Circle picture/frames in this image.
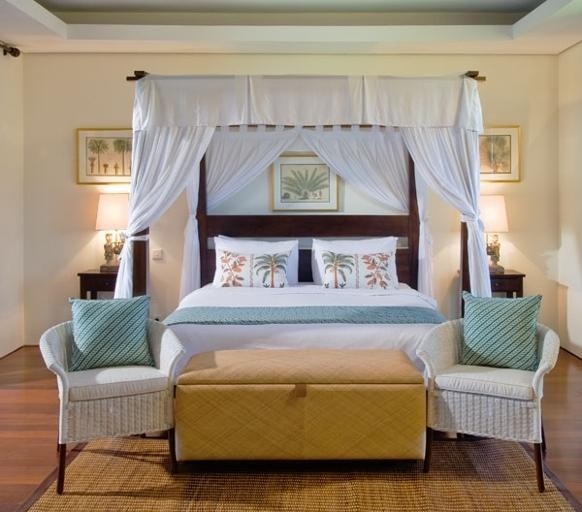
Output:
[271,155,339,212]
[479,125,521,183]
[77,128,134,185]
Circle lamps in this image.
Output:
[95,193,130,274]
[478,195,509,273]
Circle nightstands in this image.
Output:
[455,269,526,299]
[77,268,118,299]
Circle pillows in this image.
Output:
[456,290,543,371]
[212,235,296,288]
[312,235,398,292]
[68,295,156,373]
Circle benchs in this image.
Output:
[173,349,427,463]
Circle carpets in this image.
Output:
[16,436,582,512]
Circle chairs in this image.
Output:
[415,318,561,493]
[39,319,186,495]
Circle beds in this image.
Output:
[112,72,492,440]
